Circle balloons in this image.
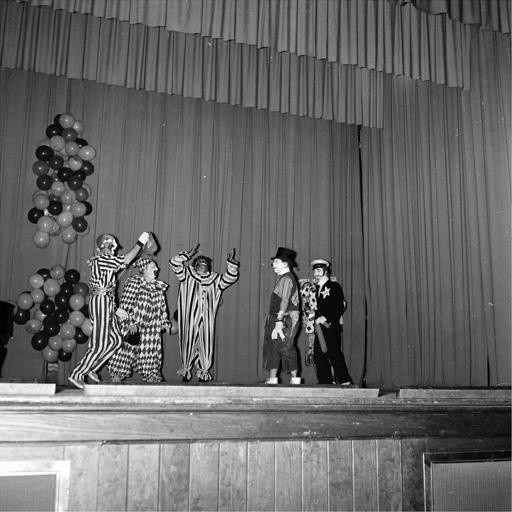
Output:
[28,112,96,249]
[9,265,92,363]
[173,308,179,320]
[165,318,178,334]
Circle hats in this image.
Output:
[310,260,331,271]
[270,246,299,268]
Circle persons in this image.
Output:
[169,243,240,383]
[68,231,150,389]
[108,258,171,383]
[310,258,352,386]
[262,246,303,385]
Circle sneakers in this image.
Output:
[257,377,280,387]
[68,377,86,389]
[198,376,208,386]
[181,372,191,385]
[88,371,100,383]
[289,377,302,385]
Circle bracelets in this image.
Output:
[277,319,283,322]
[136,241,143,247]
